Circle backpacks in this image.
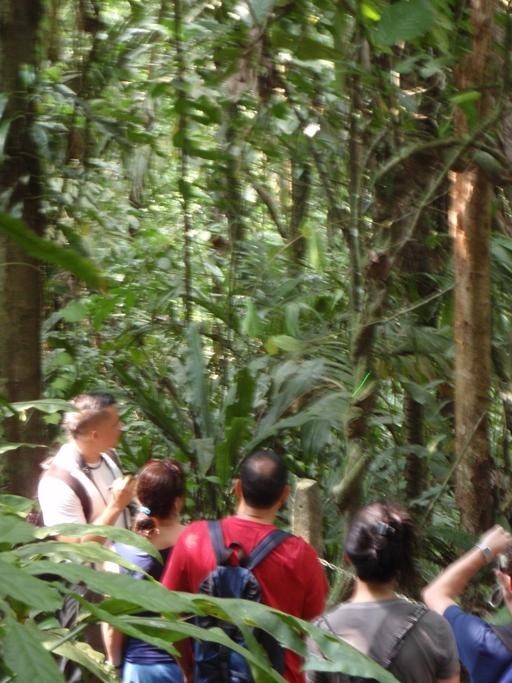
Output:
[313,604,427,683]
[21,466,90,526]
[186,520,295,683]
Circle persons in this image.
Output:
[37,392,136,683]
[159,452,331,683]
[420,524,512,683]
[304,504,459,683]
[99,459,203,683]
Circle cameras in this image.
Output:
[497,549,512,574]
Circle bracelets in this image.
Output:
[104,660,123,669]
[475,542,494,565]
